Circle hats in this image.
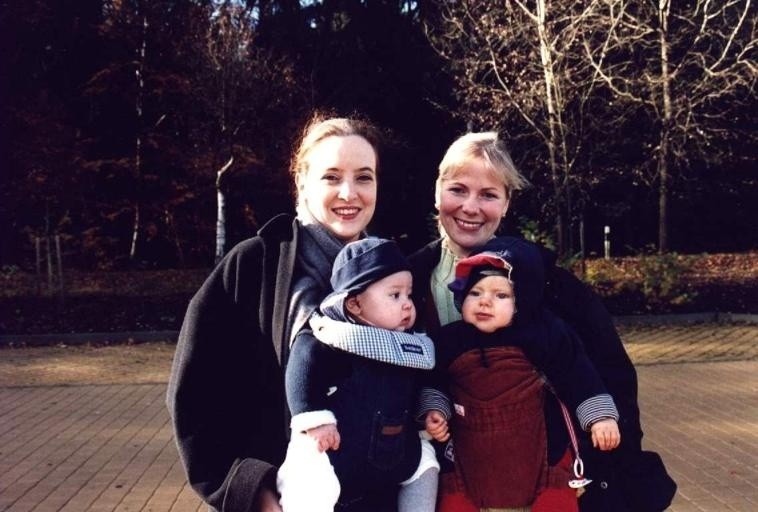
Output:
[329,237,414,294]
[447,250,525,314]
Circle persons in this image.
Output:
[275,236,444,512]
[402,131,679,512]
[166,117,394,512]
[414,234,622,512]
